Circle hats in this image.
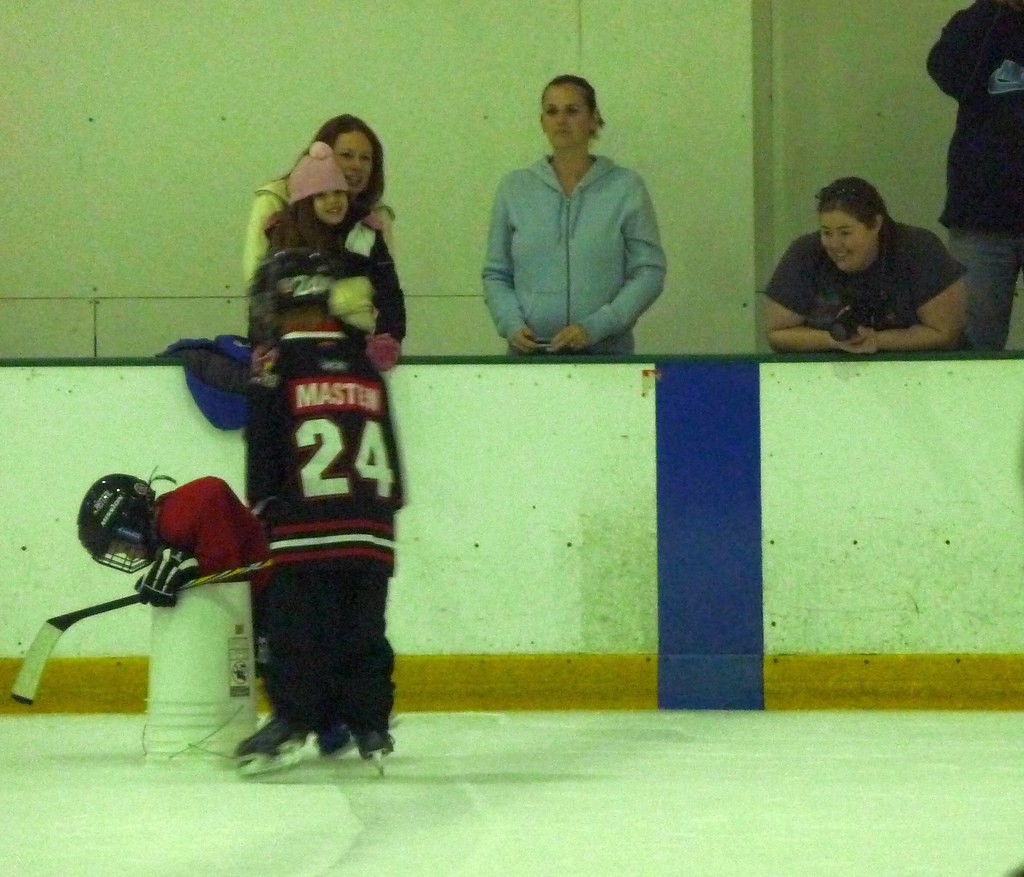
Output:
[289,140,349,205]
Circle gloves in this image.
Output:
[134,545,199,606]
[364,333,401,372]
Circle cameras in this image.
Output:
[828,300,877,341]
[531,336,554,352]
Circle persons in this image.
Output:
[926,0,1024,352]
[481,75,666,357]
[77,115,407,755]
[762,177,965,355]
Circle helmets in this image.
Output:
[248,243,331,340]
[77,474,160,573]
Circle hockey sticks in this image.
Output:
[8,558,275,707]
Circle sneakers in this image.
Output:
[235,685,323,774]
[332,697,394,777]
[316,722,354,763]
